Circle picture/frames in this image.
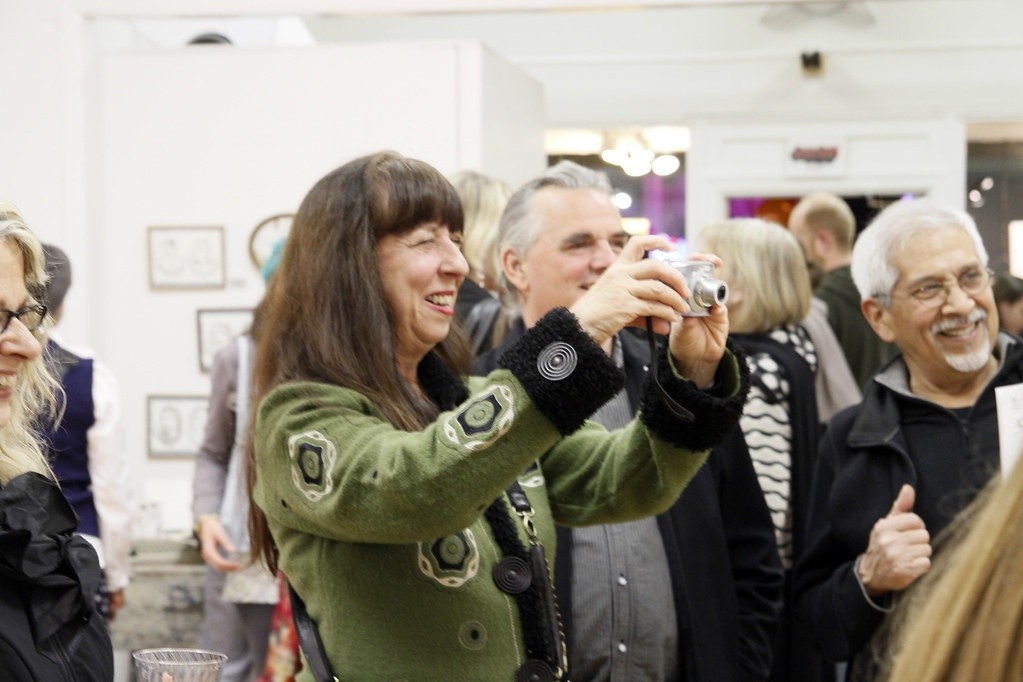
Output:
[250,212,299,274]
[196,307,257,373]
[146,392,212,459]
[146,225,228,292]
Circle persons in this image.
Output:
[461,168,784,682]
[784,198,1023,682]
[244,152,753,682]
[194,236,287,682]
[687,192,903,682]
[25,240,128,618]
[992,274,1023,342]
[0,202,116,682]
[449,172,525,352]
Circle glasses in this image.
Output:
[871,267,994,310]
[0,305,47,334]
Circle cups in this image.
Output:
[132,647,228,682]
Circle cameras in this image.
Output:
[664,260,729,318]
